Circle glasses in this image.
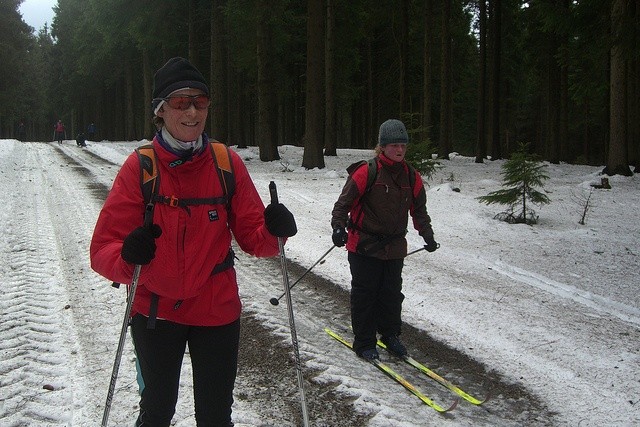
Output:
[151,94,212,109]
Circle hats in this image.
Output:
[150,58,213,116]
[378,119,409,146]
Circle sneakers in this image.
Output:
[380,335,407,356]
[352,342,379,360]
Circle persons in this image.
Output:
[75,130,88,146]
[52,118,65,145]
[87,121,98,140]
[16,120,27,142]
[90,53,299,426]
[330,117,438,360]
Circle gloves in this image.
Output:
[422,225,437,252]
[332,224,348,247]
[122,224,161,264]
[263,203,298,237]
